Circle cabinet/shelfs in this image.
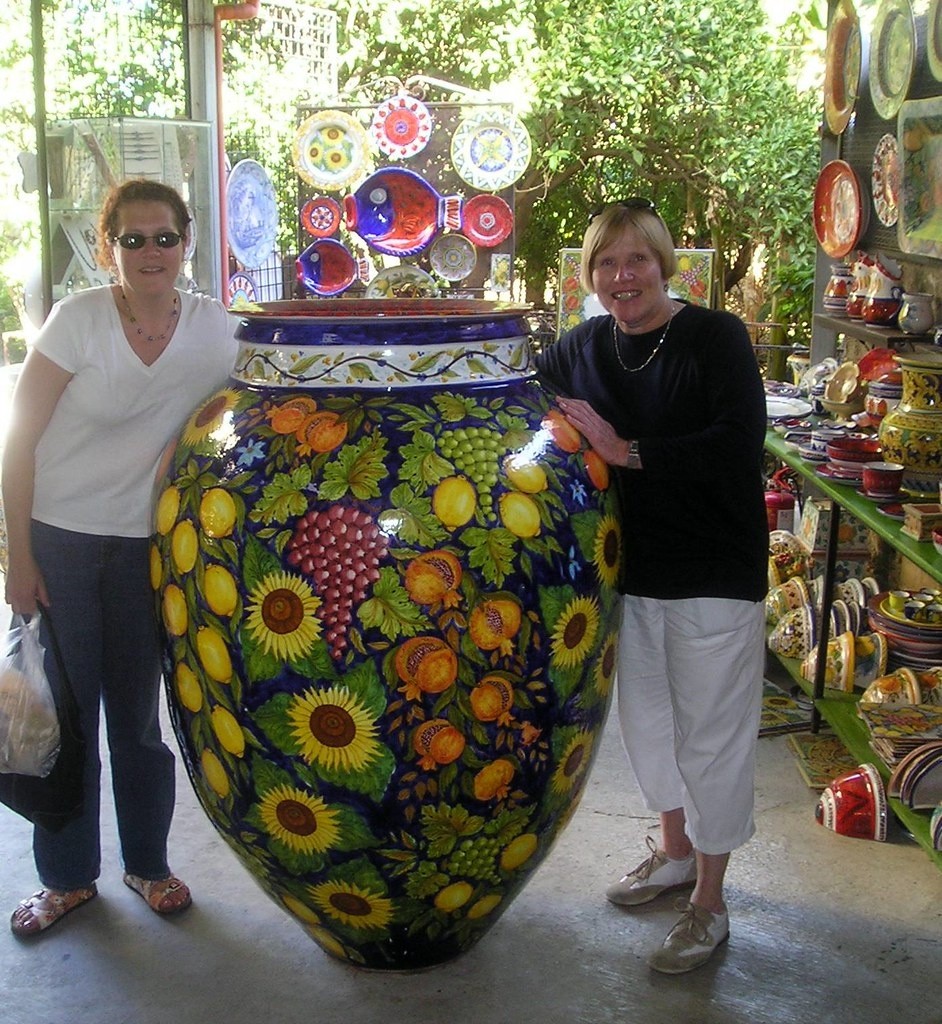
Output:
[763,310,942,869]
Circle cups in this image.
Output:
[926,604,942,624]
[922,589,940,596]
[912,593,934,605]
[888,591,910,611]
[903,601,926,621]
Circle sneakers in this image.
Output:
[604,836,701,906]
[649,904,730,974]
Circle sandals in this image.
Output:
[124,871,191,916]
[11,881,97,937]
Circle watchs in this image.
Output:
[627,440,639,469]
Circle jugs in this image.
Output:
[861,253,903,329]
[846,251,874,322]
[898,292,934,335]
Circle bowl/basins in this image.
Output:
[861,461,905,498]
[811,430,847,451]
[932,526,942,555]
[815,348,899,421]
[773,418,857,433]
[826,440,882,472]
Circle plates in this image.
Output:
[867,591,942,677]
[763,380,813,419]
[226,95,531,307]
[785,431,869,445]
[855,699,942,779]
[814,0,942,258]
[798,445,911,521]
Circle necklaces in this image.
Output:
[614,301,675,372]
[120,284,178,341]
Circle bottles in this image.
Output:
[823,263,853,319]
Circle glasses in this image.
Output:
[112,231,182,249]
[588,197,663,224]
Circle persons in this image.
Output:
[0,182,241,935]
[532,197,770,974]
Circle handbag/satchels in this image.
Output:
[0,598,83,835]
[0,609,61,779]
